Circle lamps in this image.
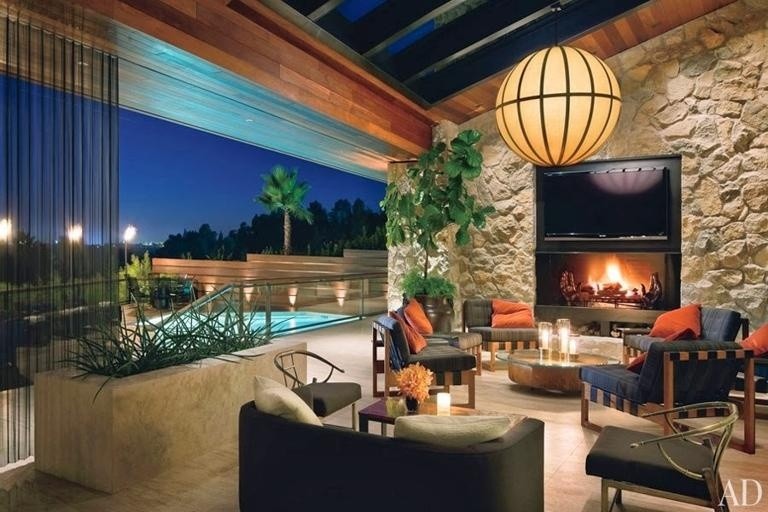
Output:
[494,0,624,168]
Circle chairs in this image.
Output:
[237,291,766,512]
[122,271,199,312]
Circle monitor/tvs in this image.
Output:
[536,154,682,252]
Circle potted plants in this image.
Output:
[32,279,308,496]
[377,127,496,335]
